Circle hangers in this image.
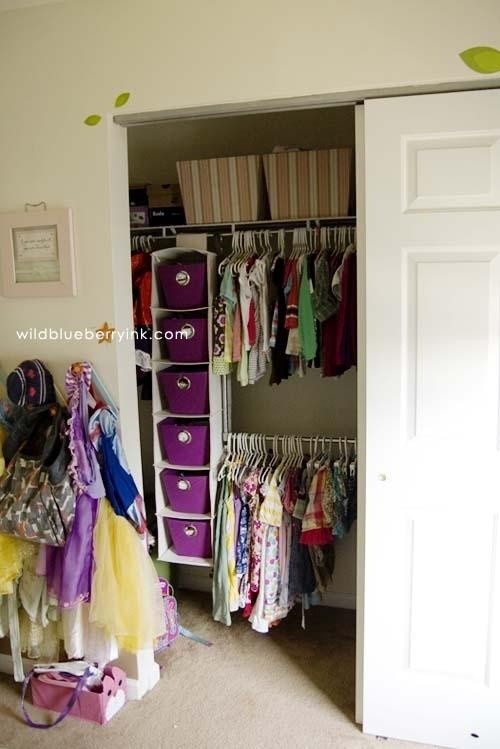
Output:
[217,433,356,488]
[218,227,357,277]
[131,235,158,253]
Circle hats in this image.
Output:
[4,358,53,408]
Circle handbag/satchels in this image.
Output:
[0,404,76,551]
[18,660,100,728]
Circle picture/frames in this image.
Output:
[1,206,77,299]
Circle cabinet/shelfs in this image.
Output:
[149,248,231,569]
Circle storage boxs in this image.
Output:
[129,147,354,227]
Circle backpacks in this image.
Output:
[153,576,180,655]
